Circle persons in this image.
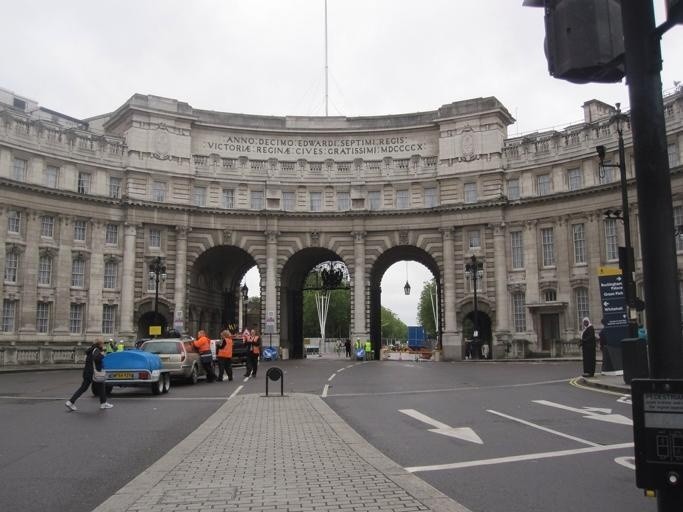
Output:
[190,329,214,383]
[242,329,259,378]
[334,339,373,361]
[214,330,233,382]
[103,337,125,354]
[577,317,594,377]
[64,337,113,411]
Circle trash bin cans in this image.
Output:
[620,338,648,386]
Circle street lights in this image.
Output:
[240,283,249,331]
[590,101,645,387]
[147,256,165,324]
[465,254,484,359]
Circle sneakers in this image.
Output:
[100,402,113,408]
[65,401,76,410]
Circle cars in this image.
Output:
[390,344,400,351]
[395,341,402,345]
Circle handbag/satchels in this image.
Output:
[93,368,105,383]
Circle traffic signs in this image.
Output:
[598,275,624,299]
[600,299,628,327]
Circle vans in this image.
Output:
[209,339,220,361]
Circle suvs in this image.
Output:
[138,335,215,384]
[217,337,247,365]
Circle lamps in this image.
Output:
[401,262,410,294]
[240,268,249,298]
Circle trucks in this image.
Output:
[407,326,427,351]
